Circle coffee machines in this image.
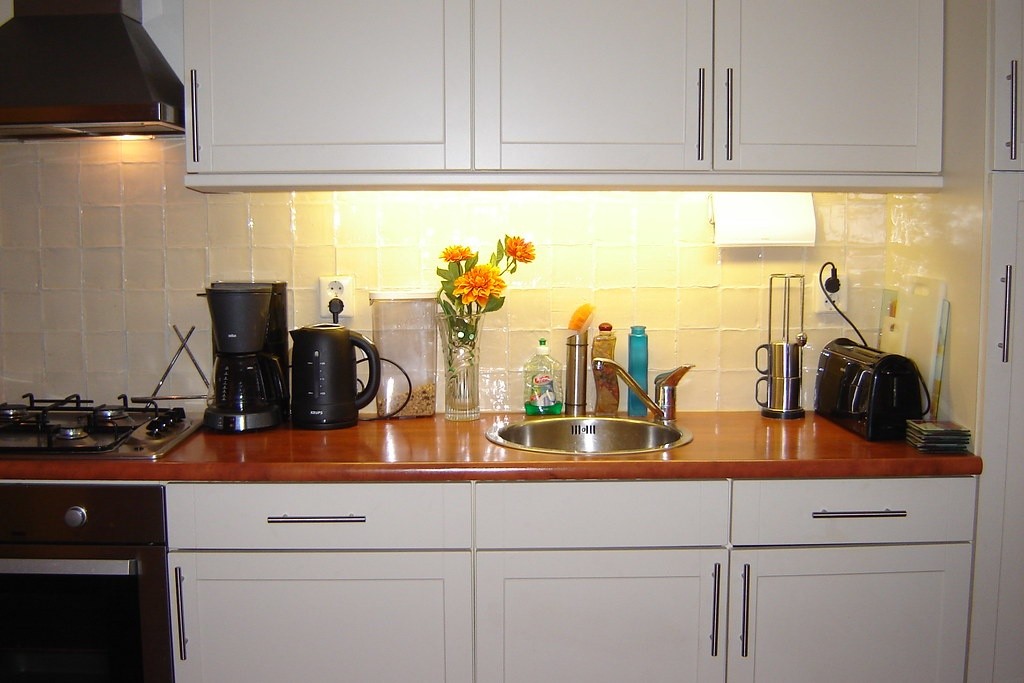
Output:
[202,279,290,433]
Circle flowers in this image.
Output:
[433,235,536,399]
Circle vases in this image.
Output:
[435,313,479,422]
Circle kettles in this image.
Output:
[288,323,382,431]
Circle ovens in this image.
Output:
[0,480,176,683]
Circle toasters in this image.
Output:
[812,337,926,440]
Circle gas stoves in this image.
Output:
[0,394,205,459]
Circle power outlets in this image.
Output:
[815,274,848,314]
[319,276,356,317]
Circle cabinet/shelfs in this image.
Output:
[183,0,945,194]
[164,477,977,683]
[886,0,1024,683]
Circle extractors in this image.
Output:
[1,0,185,144]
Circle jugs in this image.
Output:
[208,352,284,412]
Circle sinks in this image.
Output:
[484,416,695,456]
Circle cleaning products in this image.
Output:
[592,322,620,416]
[628,325,649,417]
[524,338,563,416]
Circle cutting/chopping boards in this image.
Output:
[879,275,950,419]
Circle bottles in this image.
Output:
[628,325,648,418]
[520,324,563,416]
[590,323,621,416]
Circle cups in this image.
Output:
[369,289,442,418]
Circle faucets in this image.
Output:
[591,357,696,421]
